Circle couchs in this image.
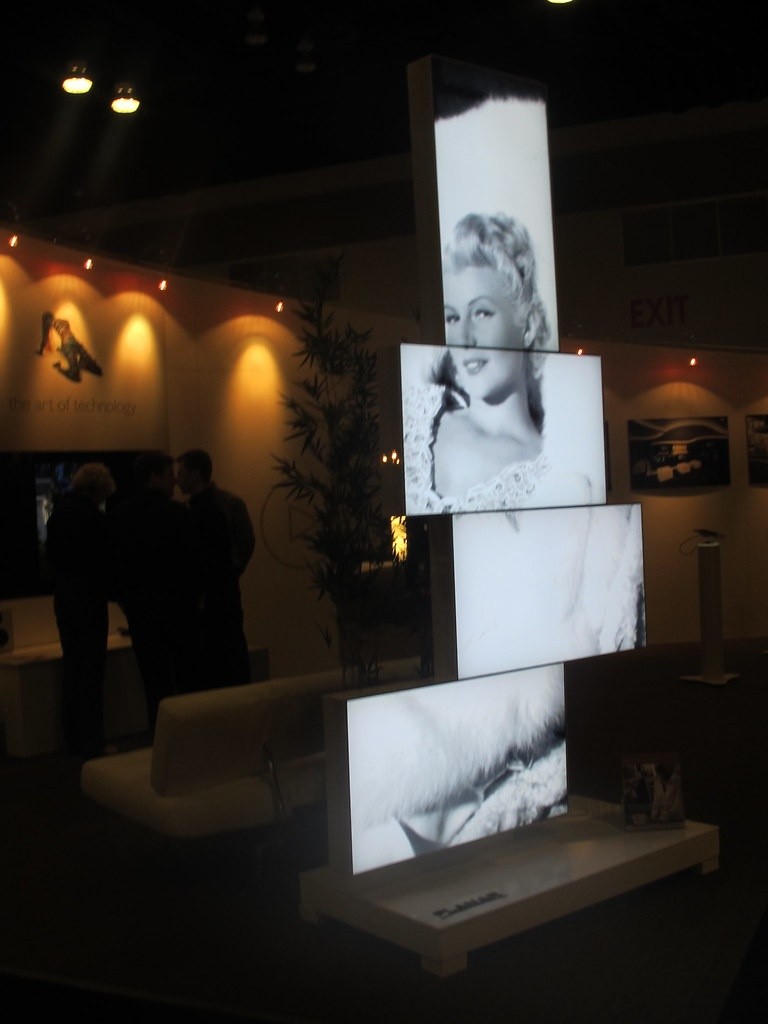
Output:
[81,650,437,861]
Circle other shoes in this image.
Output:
[59,744,85,769]
[86,743,119,758]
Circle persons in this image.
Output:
[348,212,646,820]
[110,449,256,731]
[42,464,116,760]
[623,764,683,824]
